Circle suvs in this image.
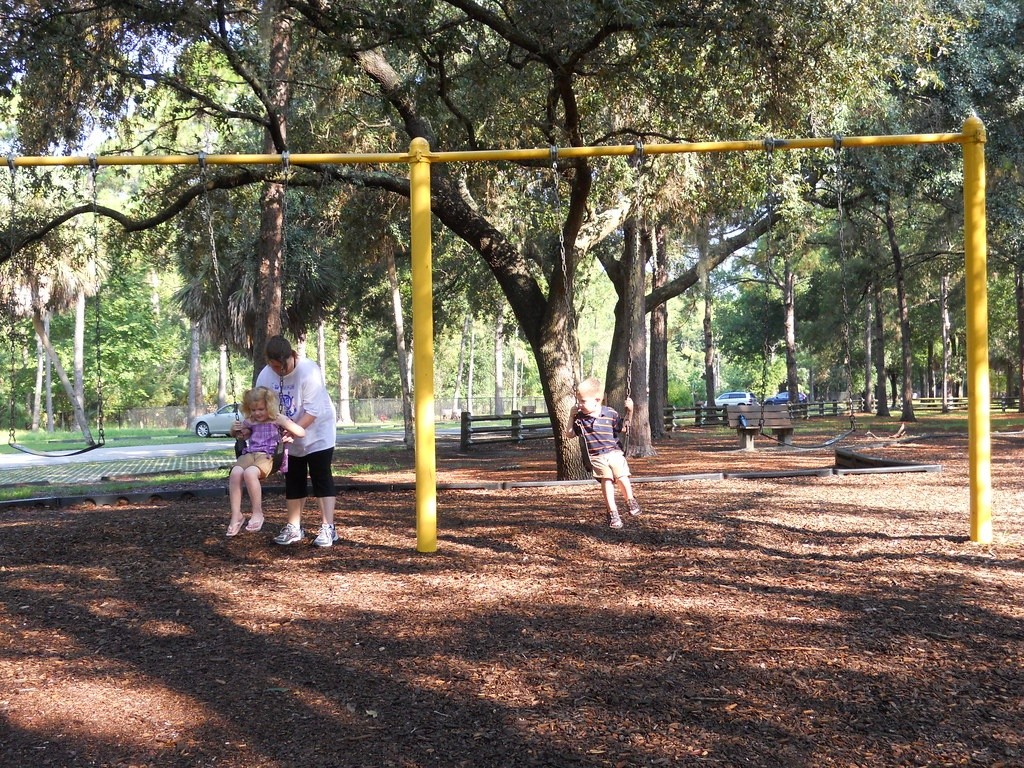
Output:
[703,391,758,411]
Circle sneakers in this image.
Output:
[312,524,338,547]
[273,523,304,545]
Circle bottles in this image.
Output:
[739,415,748,426]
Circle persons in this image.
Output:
[566,378,642,529]
[226,335,339,547]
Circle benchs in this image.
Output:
[724,404,795,449]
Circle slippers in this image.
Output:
[226,517,246,537]
[245,517,264,532]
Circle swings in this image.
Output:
[549,156,642,473]
[7,167,105,457]
[198,163,290,476]
[759,147,857,449]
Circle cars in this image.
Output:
[189,403,244,438]
[764,391,808,405]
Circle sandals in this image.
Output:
[625,499,642,517]
[608,511,623,530]
